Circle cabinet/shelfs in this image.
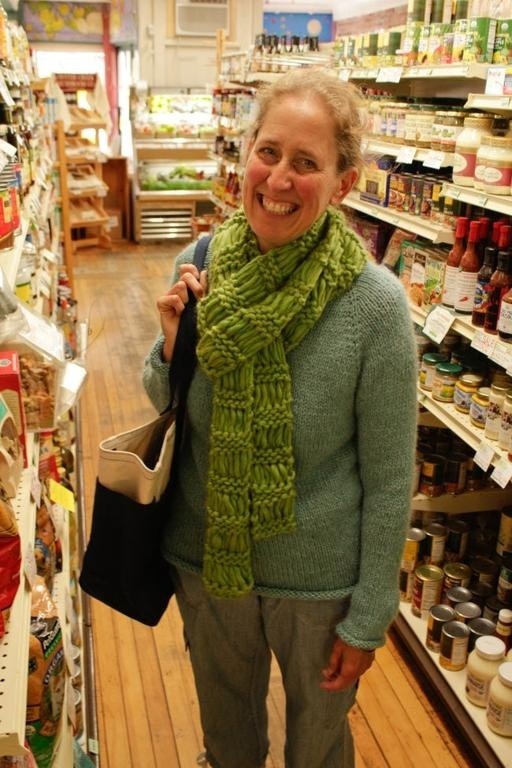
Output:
[209,60,512,768]
[128,85,213,244]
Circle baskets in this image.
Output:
[54,72,97,94]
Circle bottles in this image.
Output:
[465,634,508,708]
[486,137,512,195]
[486,661,512,737]
[209,88,253,120]
[212,163,243,208]
[448,115,495,186]
[412,214,512,343]
[251,32,321,73]
[474,135,493,189]
[494,608,512,655]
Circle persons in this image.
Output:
[138,69,419,768]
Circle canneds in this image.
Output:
[415,329,511,465]
[388,172,462,228]
[372,98,465,154]
[412,421,492,499]
[452,114,512,199]
[397,510,511,672]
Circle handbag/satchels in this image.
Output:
[78,405,189,628]
